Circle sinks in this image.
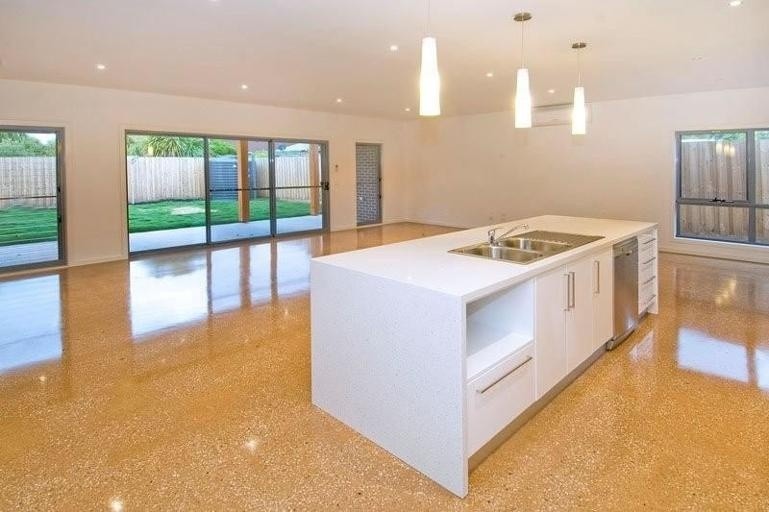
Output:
[447,243,543,265]
[495,236,571,254]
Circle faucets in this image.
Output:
[488,221,530,248]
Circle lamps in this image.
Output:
[419,0,441,116]
[571,42,587,136]
[514,12,532,128]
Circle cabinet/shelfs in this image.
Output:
[466,225,659,479]
